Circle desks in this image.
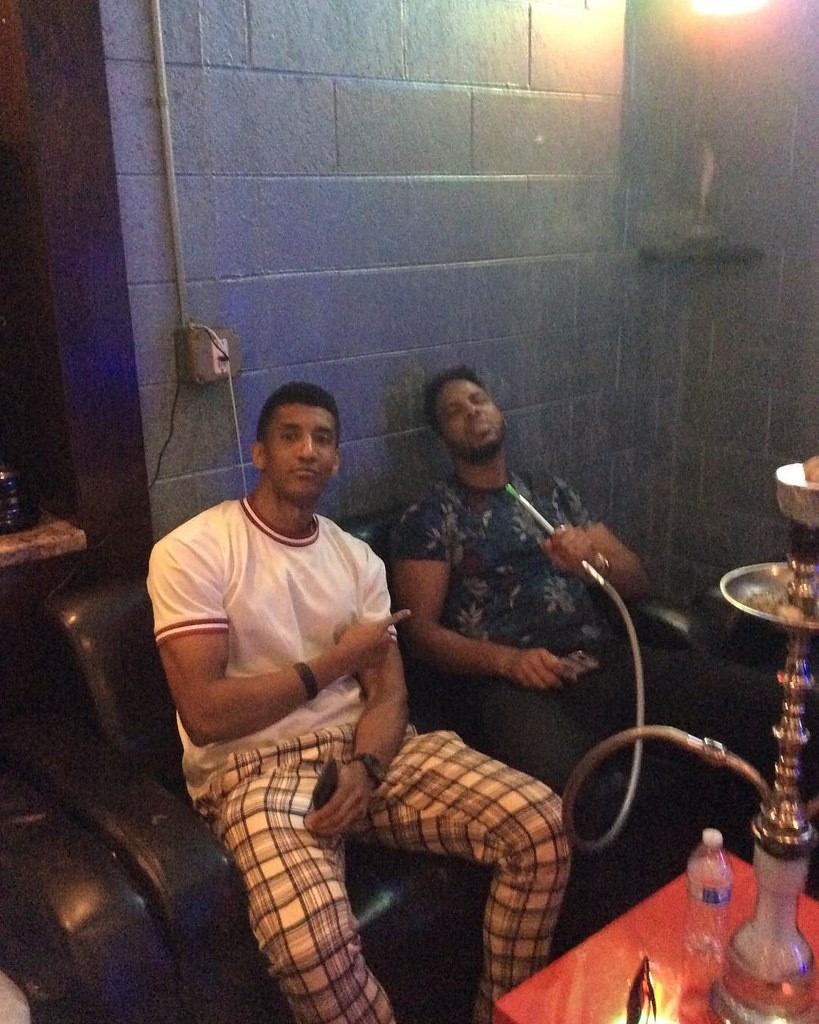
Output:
[491,842,819,1024]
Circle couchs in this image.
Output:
[337,498,716,877]
[0,553,468,1024]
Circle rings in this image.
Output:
[560,522,568,536]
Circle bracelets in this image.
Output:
[294,660,318,704]
[594,550,613,580]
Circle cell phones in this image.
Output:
[312,757,338,811]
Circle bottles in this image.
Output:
[679,827,735,959]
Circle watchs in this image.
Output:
[347,753,384,791]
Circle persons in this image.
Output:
[146,381,570,1024]
[390,366,747,924]
